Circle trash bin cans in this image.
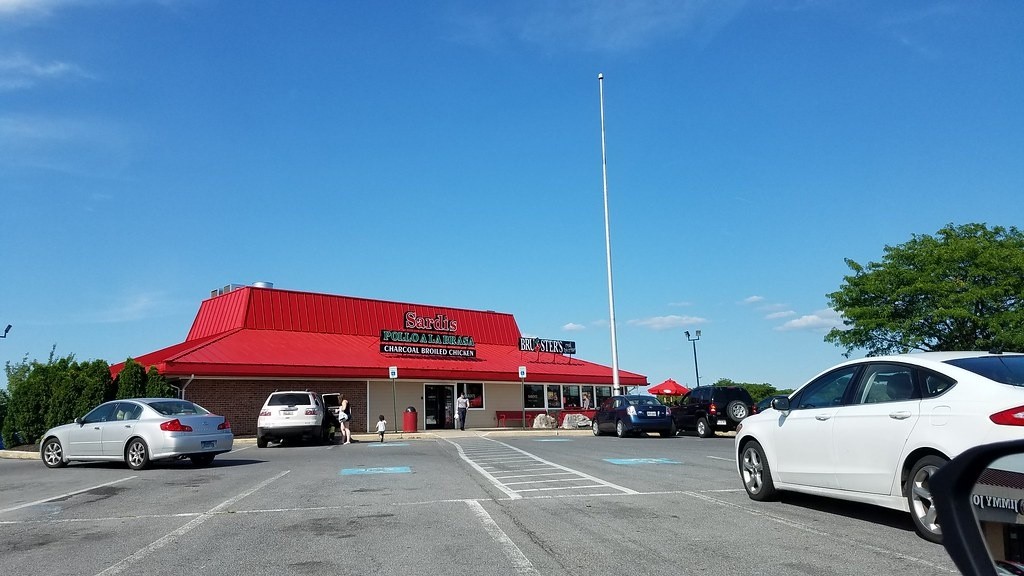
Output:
[403,407,418,433]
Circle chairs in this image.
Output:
[124,411,132,420]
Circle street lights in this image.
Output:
[683,329,701,387]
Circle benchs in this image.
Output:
[495,410,530,428]
[524,411,546,427]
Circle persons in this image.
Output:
[335,393,351,445]
[457,392,469,431]
[583,394,590,409]
[376,415,387,443]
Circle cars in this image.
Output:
[995,559,1024,576]
[592,396,672,439]
[736,351,1024,546]
[756,394,788,413]
[40,397,234,471]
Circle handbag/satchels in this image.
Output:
[338,407,348,423]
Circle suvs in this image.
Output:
[256,389,342,448]
[671,385,756,438]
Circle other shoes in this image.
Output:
[343,441,351,445]
[381,438,383,443]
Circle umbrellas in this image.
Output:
[647,377,691,407]
